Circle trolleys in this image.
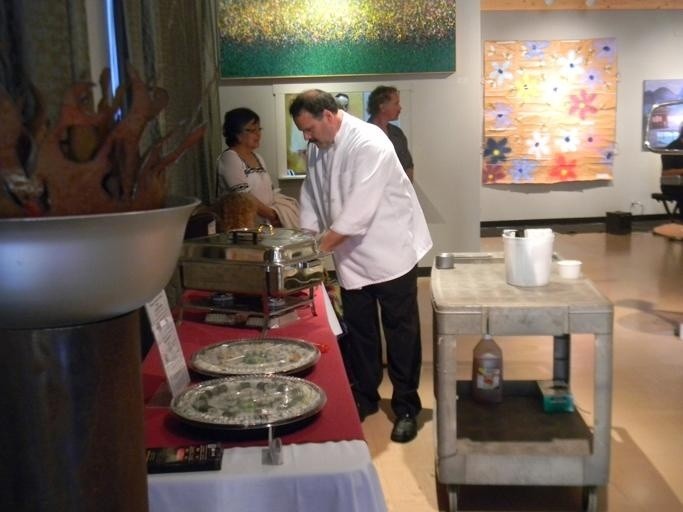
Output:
[424,252,614,512]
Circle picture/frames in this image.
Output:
[274,91,414,181]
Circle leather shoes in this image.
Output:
[392,410,418,443]
[357,404,378,423]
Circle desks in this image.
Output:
[142,283,388,512]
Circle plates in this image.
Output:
[170,377,327,430]
[187,338,322,378]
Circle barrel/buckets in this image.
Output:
[606,211,632,235]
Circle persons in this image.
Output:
[367,86,414,183]
[215,106,281,229]
[289,89,433,443]
[661,123,683,218]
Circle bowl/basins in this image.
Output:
[0,196,204,335]
[435,252,454,269]
[558,259,581,279]
